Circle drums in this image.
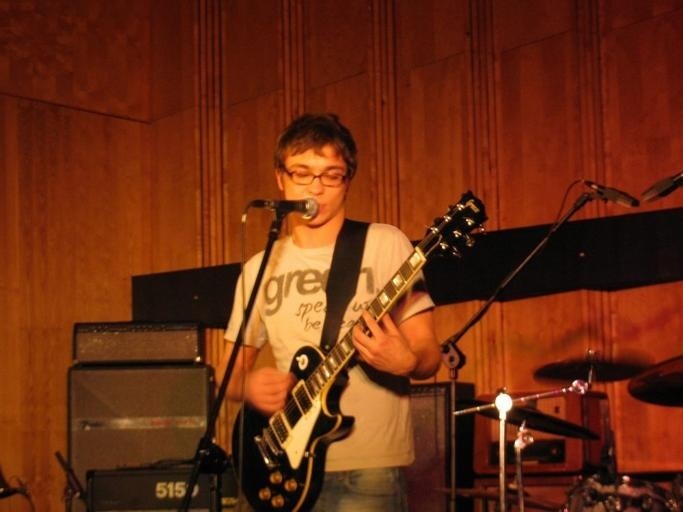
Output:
[560,476,679,511]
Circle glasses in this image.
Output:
[278,160,348,188]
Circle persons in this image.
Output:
[209,111,446,512]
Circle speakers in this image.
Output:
[68,366,216,512]
[408,382,474,512]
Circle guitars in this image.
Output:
[232,190,489,512]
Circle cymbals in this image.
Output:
[455,398,601,442]
[626,354,682,410]
[534,360,644,383]
[433,484,563,512]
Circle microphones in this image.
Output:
[584,180,640,208]
[250,199,318,217]
